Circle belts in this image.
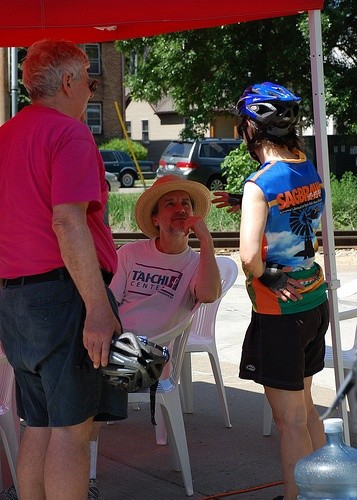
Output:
[2,266,115,286]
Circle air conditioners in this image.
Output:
[91,125,101,134]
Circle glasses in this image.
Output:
[236,118,254,137]
[70,73,99,92]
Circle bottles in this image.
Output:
[293,417,357,500]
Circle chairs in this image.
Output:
[154,256,238,448]
[262,277,357,449]
[86,297,202,495]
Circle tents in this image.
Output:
[0,0,351,450]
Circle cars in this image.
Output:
[105,172,122,192]
[99,150,156,187]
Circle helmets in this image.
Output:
[97,332,170,393]
[235,82,303,137]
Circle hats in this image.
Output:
[135,174,212,239]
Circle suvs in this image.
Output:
[156,138,244,191]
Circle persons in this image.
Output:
[212,83,332,500]
[107,174,223,343]
[0,37,129,500]
